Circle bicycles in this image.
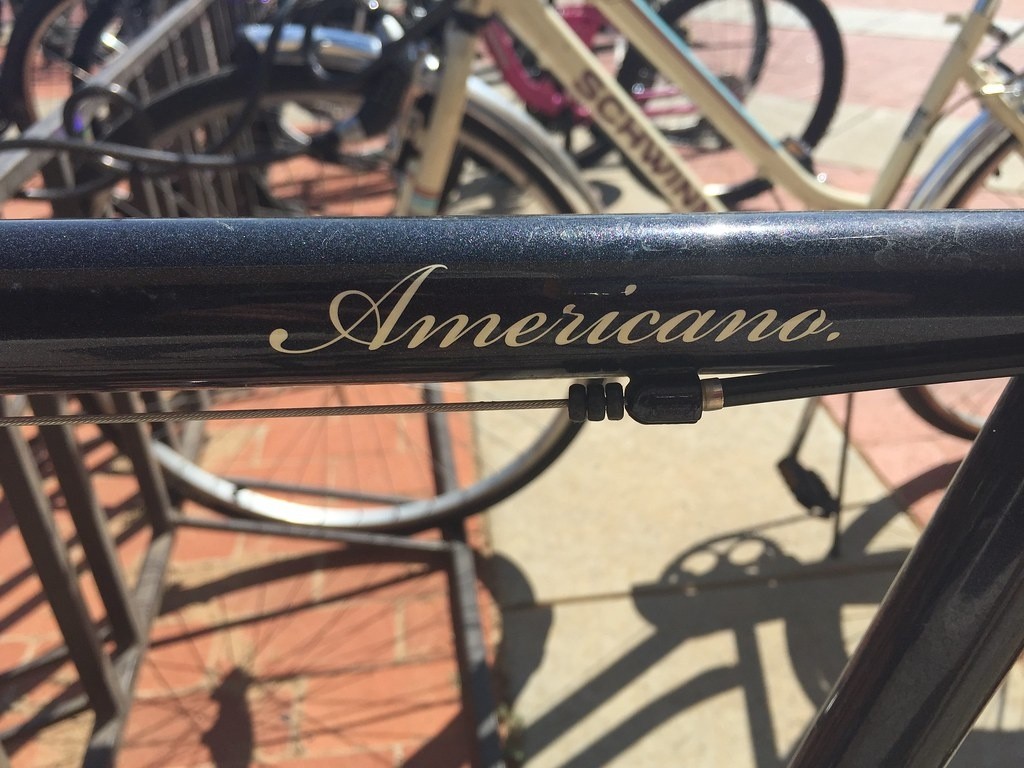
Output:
[0,0,1020,535]
